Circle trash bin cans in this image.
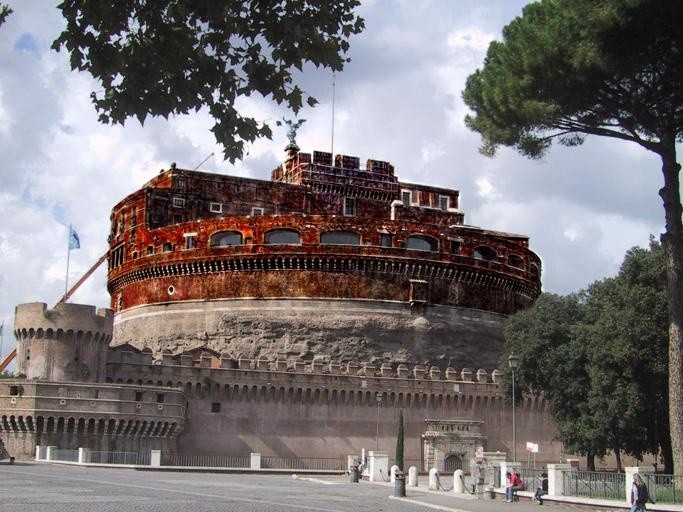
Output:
[350,465,358,482]
[395,472,405,496]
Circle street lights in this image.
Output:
[374,391,383,452]
[508,350,518,465]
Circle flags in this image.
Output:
[69,229,80,250]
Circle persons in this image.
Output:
[357,462,363,479]
[631,473,648,512]
[501,472,524,502]
[531,473,548,505]
